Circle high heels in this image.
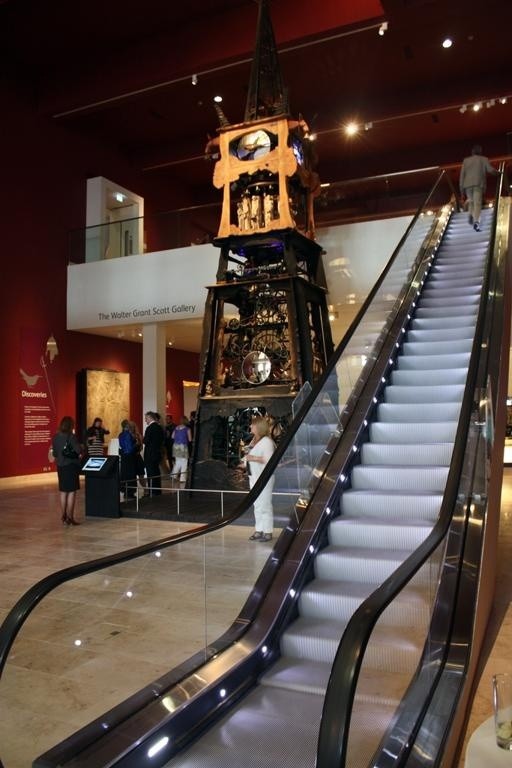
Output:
[60,512,80,526]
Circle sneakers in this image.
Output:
[248,530,273,542]
[468,214,481,232]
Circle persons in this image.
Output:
[459,144,504,231]
[51,411,196,526]
[245,416,277,542]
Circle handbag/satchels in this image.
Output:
[62,433,79,459]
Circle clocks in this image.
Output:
[238,127,270,161]
